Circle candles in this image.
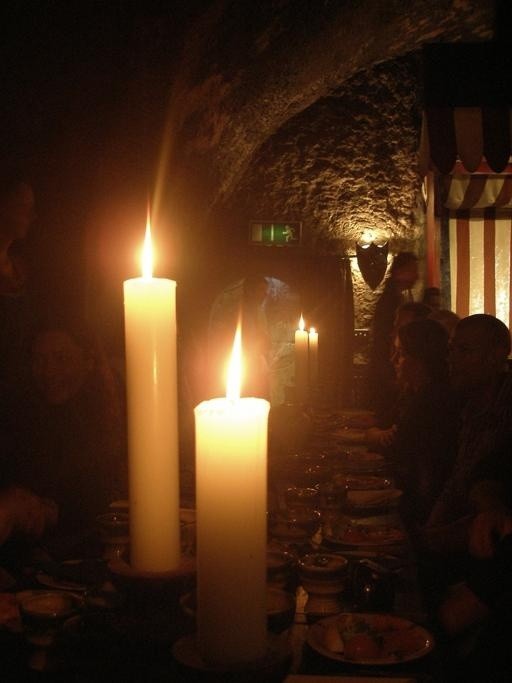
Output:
[122,273,186,581]
[186,393,275,673]
[295,313,318,403]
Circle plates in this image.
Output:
[304,611,435,666]
[266,433,418,630]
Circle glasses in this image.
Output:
[391,349,417,357]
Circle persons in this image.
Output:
[0,167,154,681]
[206,257,303,430]
[360,249,510,682]
